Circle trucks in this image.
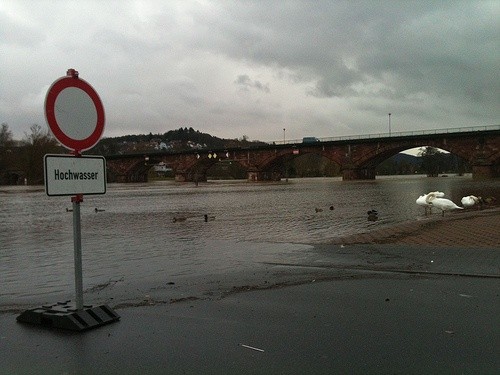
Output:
[303,137,321,143]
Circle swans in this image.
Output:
[415,191,445,216]
[461,195,480,211]
[425,192,464,217]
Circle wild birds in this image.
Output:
[368,210,378,214]
[314,208,324,213]
[65,208,73,212]
[171,218,187,222]
[95,208,105,213]
[330,206,336,210]
[204,213,215,218]
